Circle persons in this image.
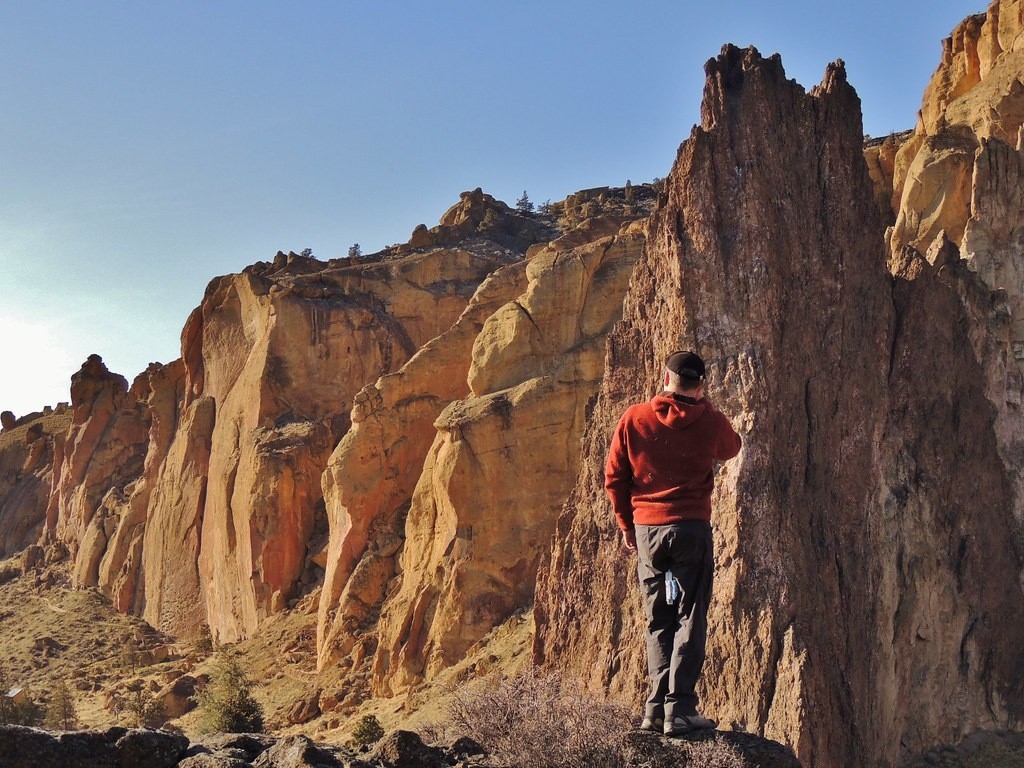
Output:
[605,350,741,737]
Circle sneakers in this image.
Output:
[640,715,663,731]
[664,714,717,735]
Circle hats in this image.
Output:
[666,351,705,380]
